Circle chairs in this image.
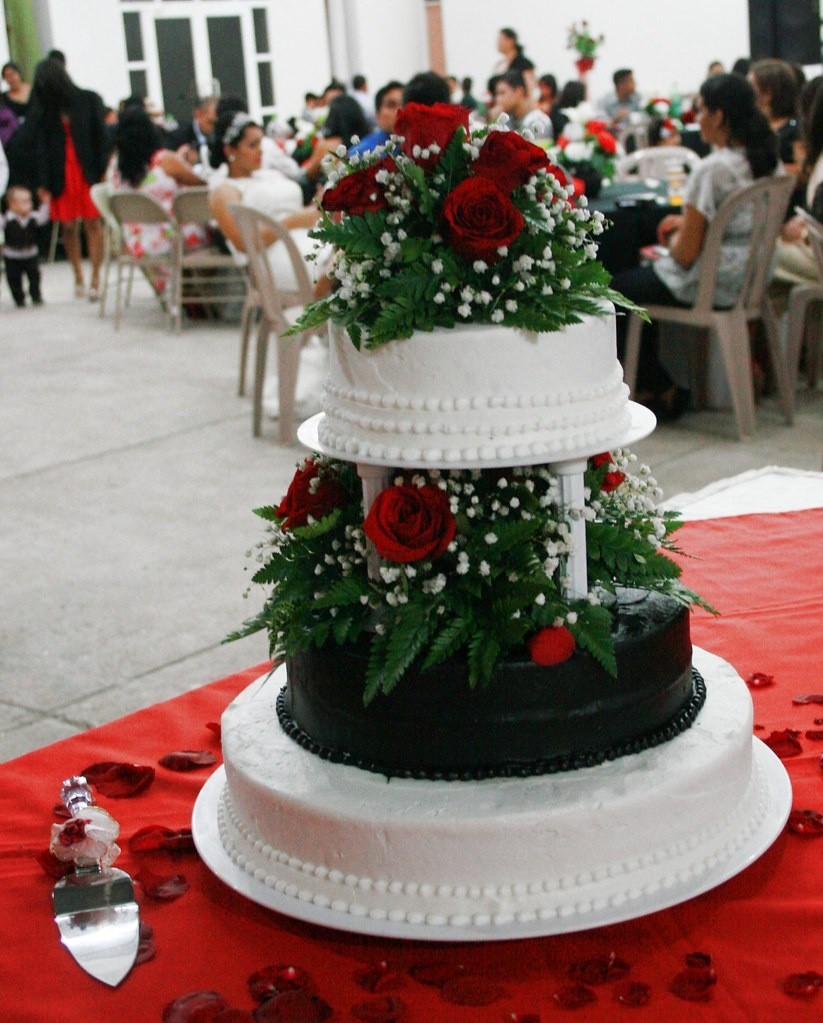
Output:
[90,182,331,445]
[624,173,823,443]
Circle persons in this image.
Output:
[497,29,535,98]
[106,59,823,421]
[0,184,51,308]
[0,50,114,301]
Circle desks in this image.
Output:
[589,178,686,382]
[0,465,823,1023]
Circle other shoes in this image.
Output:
[642,387,690,421]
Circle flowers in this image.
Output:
[568,21,604,58]
[275,101,652,355]
[220,447,721,706]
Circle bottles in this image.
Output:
[666,156,685,204]
